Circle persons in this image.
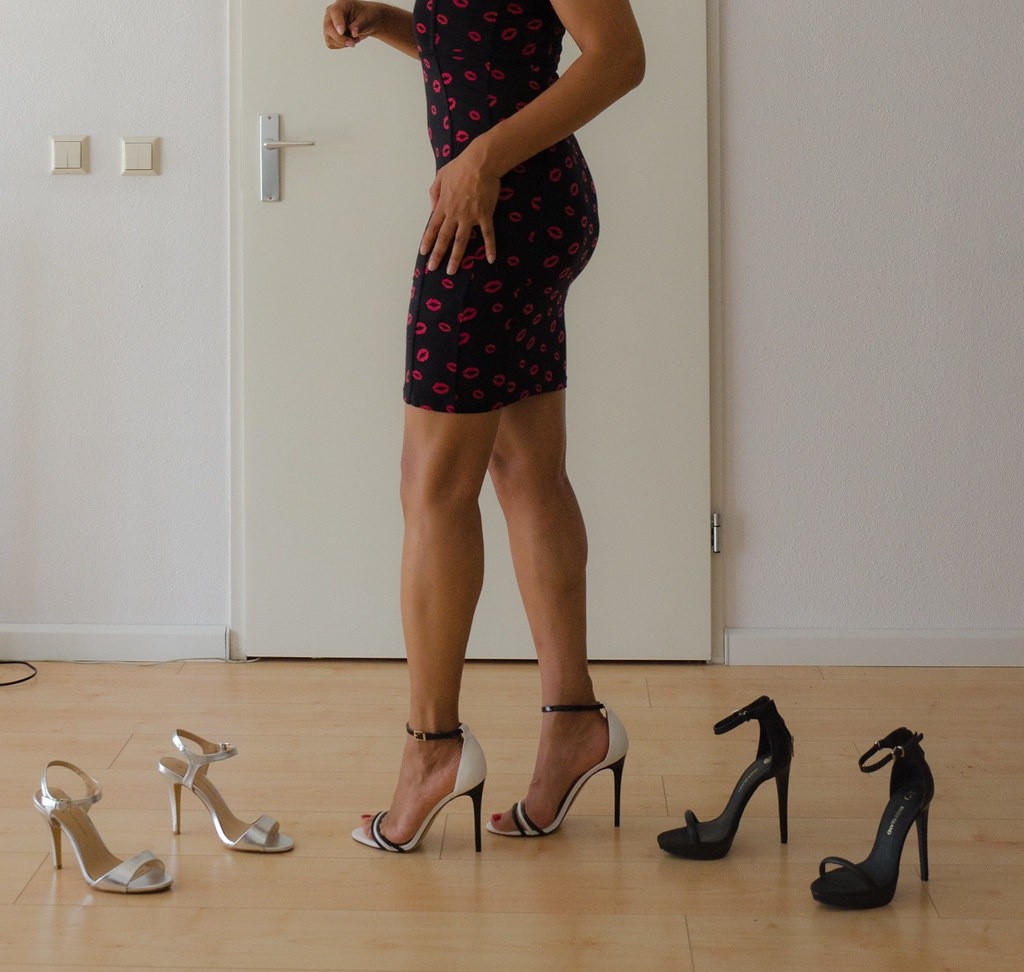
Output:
[321,0,647,854]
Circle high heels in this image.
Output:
[487,700,628,838]
[351,721,487,853]
[157,726,295,852]
[656,694,793,861]
[32,759,173,893]
[810,726,935,910]
